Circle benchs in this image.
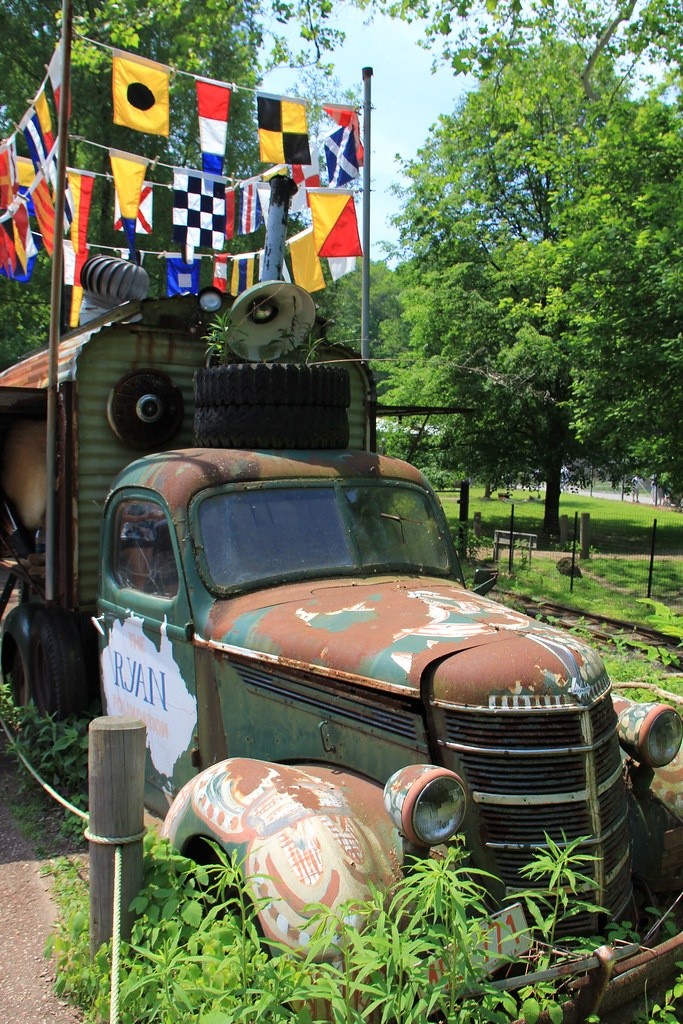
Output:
[493,528,539,566]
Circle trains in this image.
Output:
[1,268,683,1024]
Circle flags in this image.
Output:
[225,144,319,240]
[281,225,327,293]
[305,186,363,281]
[111,49,172,136]
[319,106,366,187]
[170,166,226,249]
[0,38,96,328]
[255,90,312,165]
[165,253,204,298]
[228,251,266,297]
[114,178,154,235]
